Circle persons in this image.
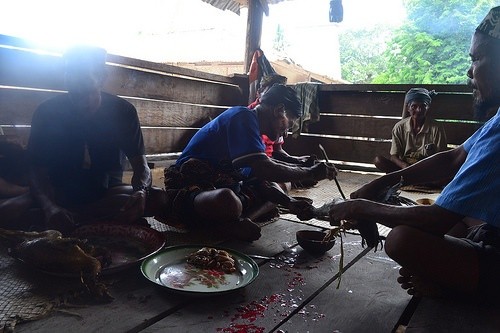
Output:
[0,44,167,230]
[329,6,500,295]
[245,73,303,164]
[163,82,337,242]
[374,88,447,174]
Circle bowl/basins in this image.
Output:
[296,230,336,253]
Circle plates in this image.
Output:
[8,220,166,277]
[140,244,260,295]
[279,197,312,212]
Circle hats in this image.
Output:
[474,5,500,41]
